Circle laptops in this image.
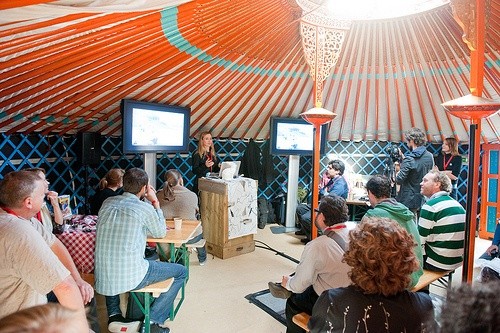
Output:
[209,160,241,178]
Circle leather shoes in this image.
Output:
[107,314,142,333]
[139,323,170,333]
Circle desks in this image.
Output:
[346,199,371,221]
[54,214,201,273]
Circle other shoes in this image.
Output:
[301,238,310,244]
[268,281,293,300]
[199,260,205,265]
[296,230,306,235]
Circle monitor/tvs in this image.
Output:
[270,115,327,156]
[120,99,191,153]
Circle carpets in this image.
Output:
[244,289,288,326]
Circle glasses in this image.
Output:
[314,208,323,214]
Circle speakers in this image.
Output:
[78,132,101,164]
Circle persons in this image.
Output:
[0,172,94,318]
[193,132,220,194]
[90,168,125,215]
[308,216,435,333]
[24,168,66,235]
[95,168,188,333]
[269,195,359,333]
[438,219,500,333]
[417,170,466,293]
[360,175,424,287]
[434,137,462,199]
[0,304,89,333]
[157,169,207,265]
[394,128,435,211]
[296,160,348,242]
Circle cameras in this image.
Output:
[385,145,400,159]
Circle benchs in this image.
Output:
[291,270,455,333]
[175,238,206,247]
[80,274,174,333]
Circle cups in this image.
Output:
[174,218,182,230]
[349,193,354,201]
